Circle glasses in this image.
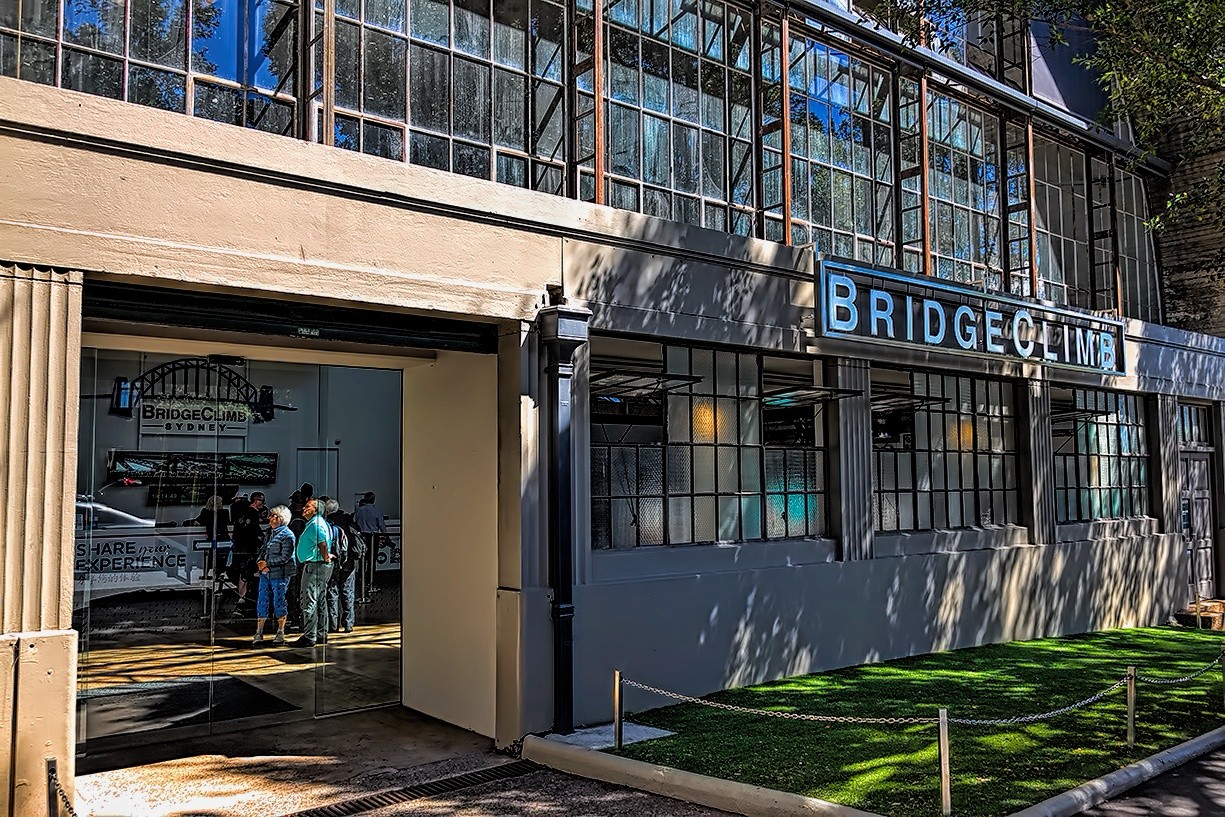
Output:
[257,499,265,502]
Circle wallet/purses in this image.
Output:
[346,524,368,559]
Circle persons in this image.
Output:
[252,506,296,643]
[287,499,337,647]
[195,483,390,634]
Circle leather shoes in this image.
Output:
[289,639,316,647]
[317,638,328,645]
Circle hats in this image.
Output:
[232,491,249,499]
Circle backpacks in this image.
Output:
[331,521,351,569]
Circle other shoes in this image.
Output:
[345,625,354,633]
[272,634,284,644]
[253,633,263,644]
